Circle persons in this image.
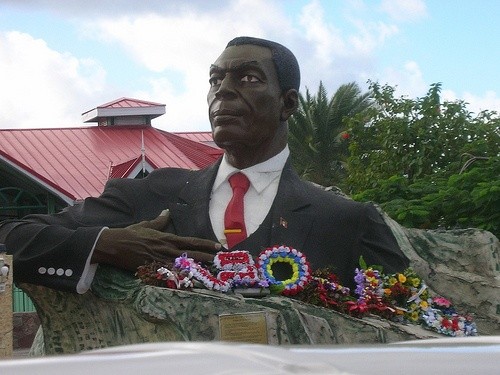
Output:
[0,36,407,313]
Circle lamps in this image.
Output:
[0,258,9,292]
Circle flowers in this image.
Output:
[306,259,478,337]
